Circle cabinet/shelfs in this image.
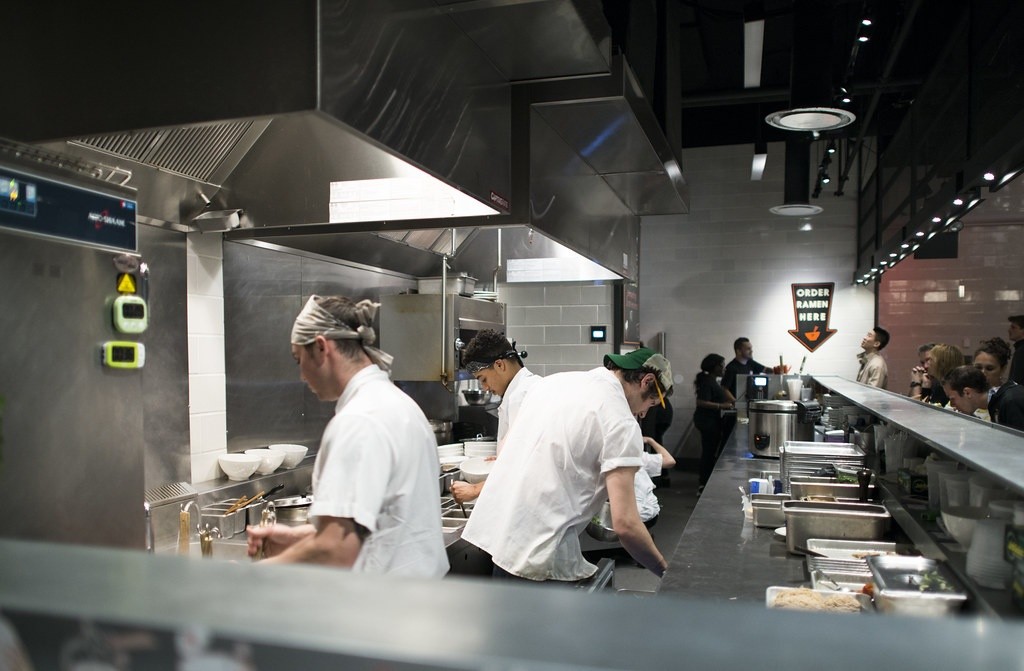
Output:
[807,374,1024,621]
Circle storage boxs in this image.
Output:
[414,274,479,296]
[782,500,891,556]
[865,555,967,616]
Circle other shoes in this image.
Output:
[696,486,704,497]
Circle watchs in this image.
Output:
[910,382,921,387]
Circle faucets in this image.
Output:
[173,500,204,559]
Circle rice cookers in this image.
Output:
[749,398,821,461]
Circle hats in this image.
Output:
[602,348,674,394]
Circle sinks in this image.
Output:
[160,539,252,563]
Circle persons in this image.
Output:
[909,314,1024,430]
[856,325,890,390]
[449,328,543,505]
[693,354,737,498]
[721,337,788,402]
[460,349,673,584]
[633,347,676,529]
[247,293,450,579]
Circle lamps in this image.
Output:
[886,92,917,107]
[812,13,874,200]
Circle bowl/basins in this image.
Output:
[218,453,262,482]
[460,457,496,484]
[245,448,286,475]
[939,501,1024,591]
[268,443,308,471]
[464,442,498,457]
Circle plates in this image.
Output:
[472,290,498,301]
[821,394,874,433]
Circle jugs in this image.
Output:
[874,423,920,477]
[786,379,812,401]
[924,460,1013,525]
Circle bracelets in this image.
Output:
[717,402,719,410]
